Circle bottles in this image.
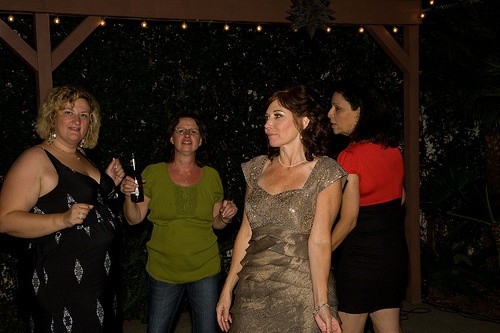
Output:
[128,153,144,203]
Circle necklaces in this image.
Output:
[47,139,81,160]
[278,154,307,168]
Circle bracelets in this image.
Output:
[221,216,232,224]
[312,302,330,318]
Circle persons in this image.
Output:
[327,78,407,333]
[216,84,348,333]
[0,85,125,333]
[120,112,238,333]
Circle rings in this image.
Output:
[78,212,83,219]
